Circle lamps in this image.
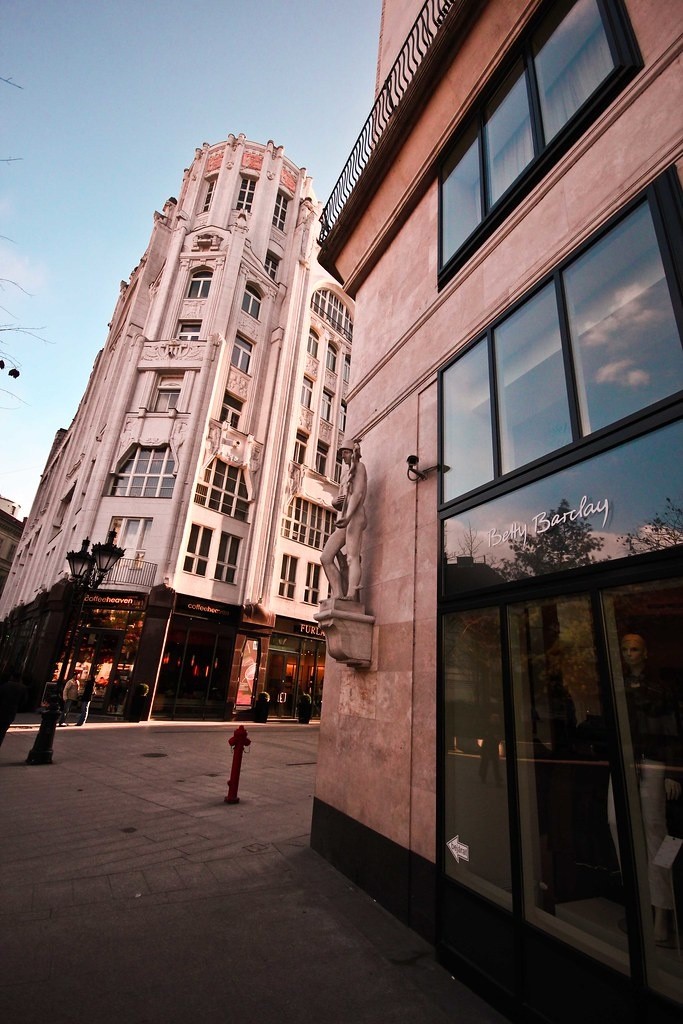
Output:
[402,454,428,482]
[257,597,263,604]
[162,577,170,587]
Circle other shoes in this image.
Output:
[59,723,67,726]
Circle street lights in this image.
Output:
[24,526,127,767]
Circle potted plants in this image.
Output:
[255,691,270,722]
[297,692,311,724]
[126,683,149,722]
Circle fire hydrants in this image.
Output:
[225,724,251,803]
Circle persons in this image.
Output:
[476,712,503,794]
[318,440,367,603]
[605,633,681,945]
[57,671,80,726]
[75,674,96,726]
